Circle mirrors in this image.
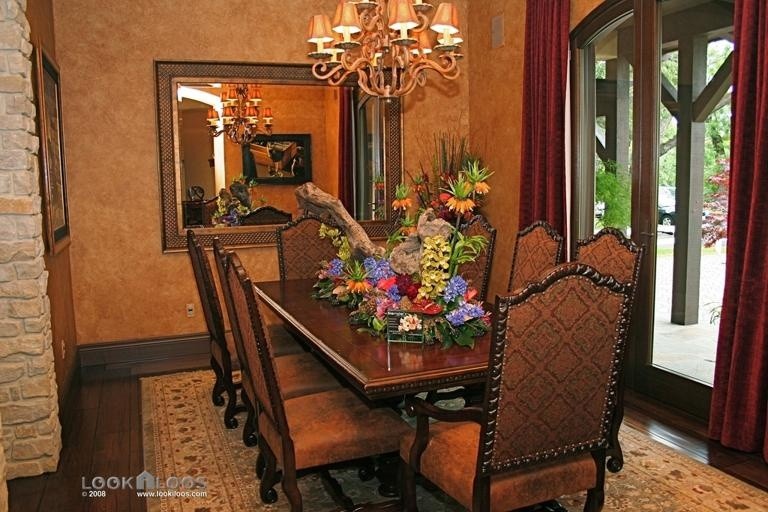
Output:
[151,58,405,254]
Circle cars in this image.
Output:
[595,186,711,235]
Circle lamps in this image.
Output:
[304,0,465,104]
[203,83,274,147]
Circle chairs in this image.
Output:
[183,216,648,512]
[239,205,292,225]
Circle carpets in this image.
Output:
[137,367,768,512]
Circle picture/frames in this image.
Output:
[240,132,314,187]
[34,40,72,255]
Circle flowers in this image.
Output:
[209,172,267,228]
[310,130,500,351]
